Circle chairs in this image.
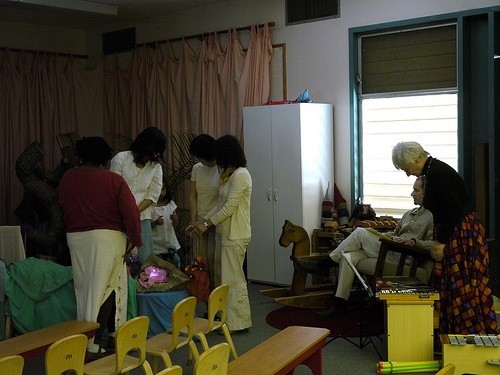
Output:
[188,284,238,358]
[0,355,25,375]
[145,296,200,375]
[83,315,154,375]
[0,226,26,339]
[156,342,230,375]
[358,216,449,295]
[44,333,88,375]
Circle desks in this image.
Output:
[135,289,190,337]
[0,320,100,364]
[384,277,434,363]
[227,326,330,375]
[441,334,500,375]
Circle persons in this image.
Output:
[56,136,142,360]
[393,141,498,335]
[187,134,224,321]
[148,179,181,270]
[185,135,253,336]
[295,176,434,321]
[107,125,166,267]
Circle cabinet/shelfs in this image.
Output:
[241,103,335,286]
[311,228,352,289]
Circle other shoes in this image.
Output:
[216,327,249,335]
[316,304,349,320]
[295,258,330,280]
[87,346,115,363]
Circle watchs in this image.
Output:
[203,221,210,229]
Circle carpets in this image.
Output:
[266,291,383,337]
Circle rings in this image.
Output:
[400,237,403,240]
